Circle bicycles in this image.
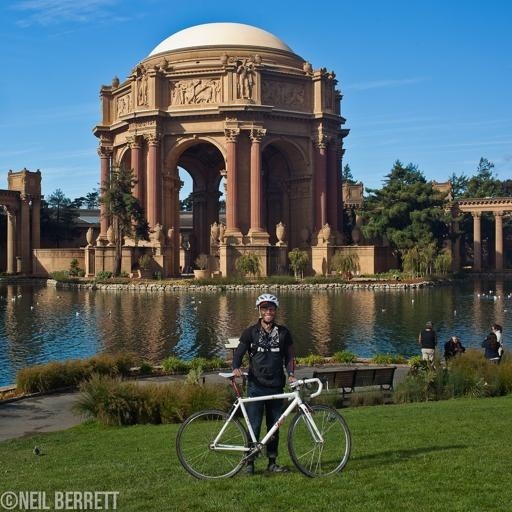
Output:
[176,372,352,480]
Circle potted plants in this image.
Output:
[334,254,359,279]
[136,256,154,280]
[287,248,310,280]
[193,252,212,281]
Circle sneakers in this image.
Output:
[246,465,253,474]
[268,463,288,473]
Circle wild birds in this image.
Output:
[190,297,202,310]
[381,308,387,311]
[411,298,414,302]
[453,310,457,315]
[33,445,40,456]
[478,290,512,312]
[3,293,65,311]
[76,311,81,317]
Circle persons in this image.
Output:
[481,332,501,364]
[419,321,437,363]
[491,324,504,360]
[232,292,297,474]
[444,336,466,360]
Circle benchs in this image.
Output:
[304,368,398,399]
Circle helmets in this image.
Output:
[256,293,279,307]
